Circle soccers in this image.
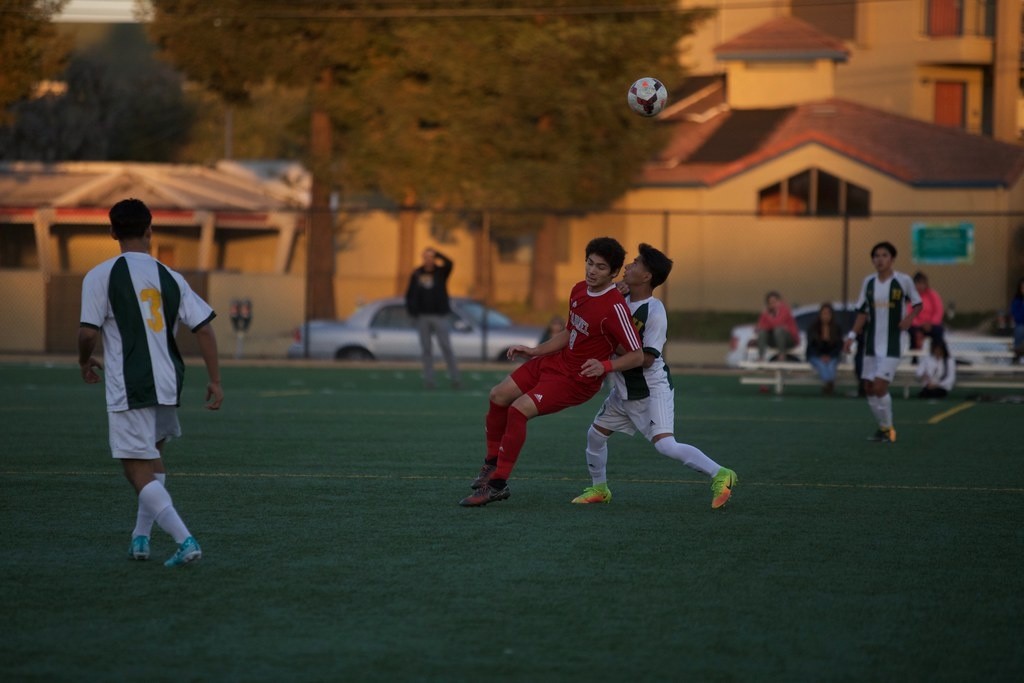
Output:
[627,77,668,118]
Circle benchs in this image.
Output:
[741,337,1024,400]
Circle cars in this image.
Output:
[286,296,545,363]
[726,300,1012,373]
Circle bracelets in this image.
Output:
[602,359,613,371]
[848,330,856,340]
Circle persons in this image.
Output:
[914,335,956,399]
[1008,278,1024,364]
[844,241,923,445]
[78,195,224,569]
[754,291,801,363]
[405,246,463,389]
[571,242,739,510]
[458,236,645,505]
[806,302,844,398]
[906,271,944,365]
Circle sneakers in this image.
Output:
[164,536,202,568]
[129,534,150,560]
[470,464,497,489]
[711,469,738,509]
[571,486,611,504]
[867,424,895,443]
[460,482,511,507]
